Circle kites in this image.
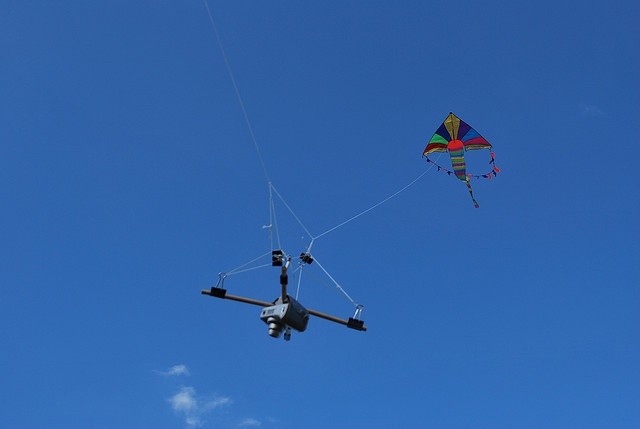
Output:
[423,111,501,208]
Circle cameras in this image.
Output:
[259,303,310,339]
